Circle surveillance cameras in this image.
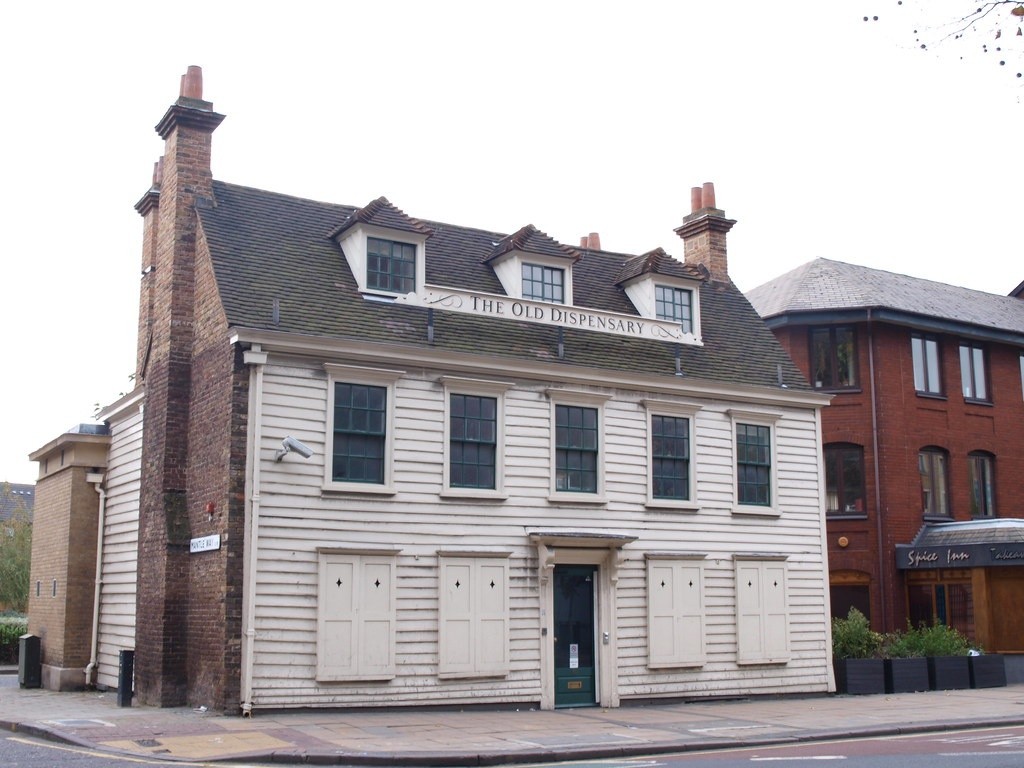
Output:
[283,436,315,458]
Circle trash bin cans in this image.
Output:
[18,634,40,688]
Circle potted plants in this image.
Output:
[831,605,1006,695]
